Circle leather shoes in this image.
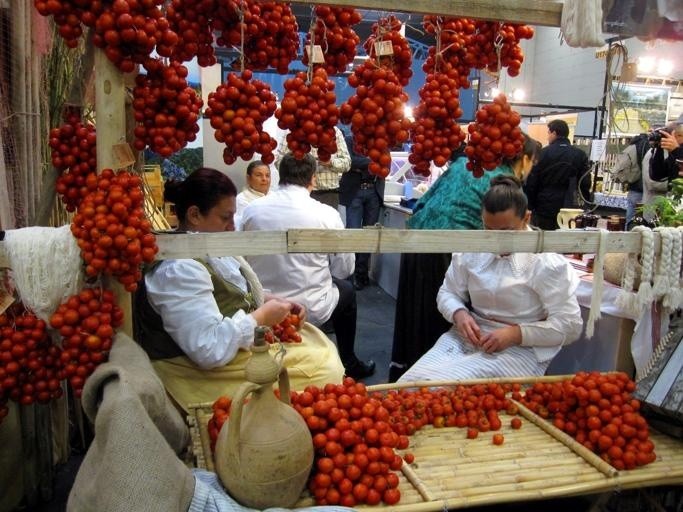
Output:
[388,364,410,381]
[358,272,369,287]
[349,274,362,290]
[344,359,375,381]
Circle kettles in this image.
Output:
[568,213,606,230]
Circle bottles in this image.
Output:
[627,203,648,231]
[213,326,314,512]
[650,202,663,229]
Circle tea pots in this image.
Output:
[557,208,585,228]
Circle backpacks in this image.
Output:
[643,149,679,192]
[612,144,641,185]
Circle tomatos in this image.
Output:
[70,169,159,293]
[265,313,302,344]
[48,107,97,213]
[0,0,534,178]
[273,371,656,508]
[208,396,249,461]
[49,287,124,399]
[0,303,65,421]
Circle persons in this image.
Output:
[648,123,682,192]
[523,119,591,231]
[387,131,542,383]
[391,174,584,394]
[609,125,655,232]
[641,127,669,229]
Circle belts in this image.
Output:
[359,183,375,190]
[312,189,339,194]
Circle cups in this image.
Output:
[607,214,625,231]
[405,179,416,198]
[404,140,412,152]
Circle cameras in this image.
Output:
[648,125,673,143]
